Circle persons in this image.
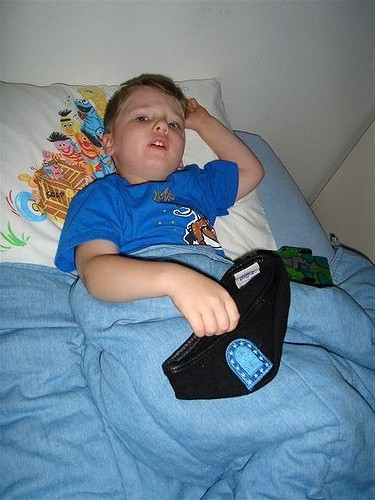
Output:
[54,73,265,338]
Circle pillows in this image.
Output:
[0,78,278,267]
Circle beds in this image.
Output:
[0,131,375,500]
